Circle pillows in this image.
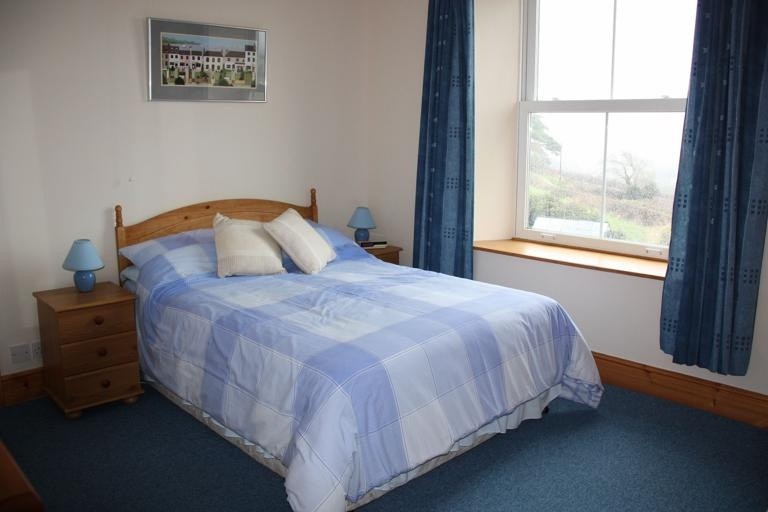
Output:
[211,207,337,278]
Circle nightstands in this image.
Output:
[32,280,146,419]
[364,245,402,264]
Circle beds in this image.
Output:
[115,189,564,512]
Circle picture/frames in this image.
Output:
[145,16,269,104]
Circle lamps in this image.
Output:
[345,207,377,244]
[63,240,106,294]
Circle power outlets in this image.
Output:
[31,339,42,358]
